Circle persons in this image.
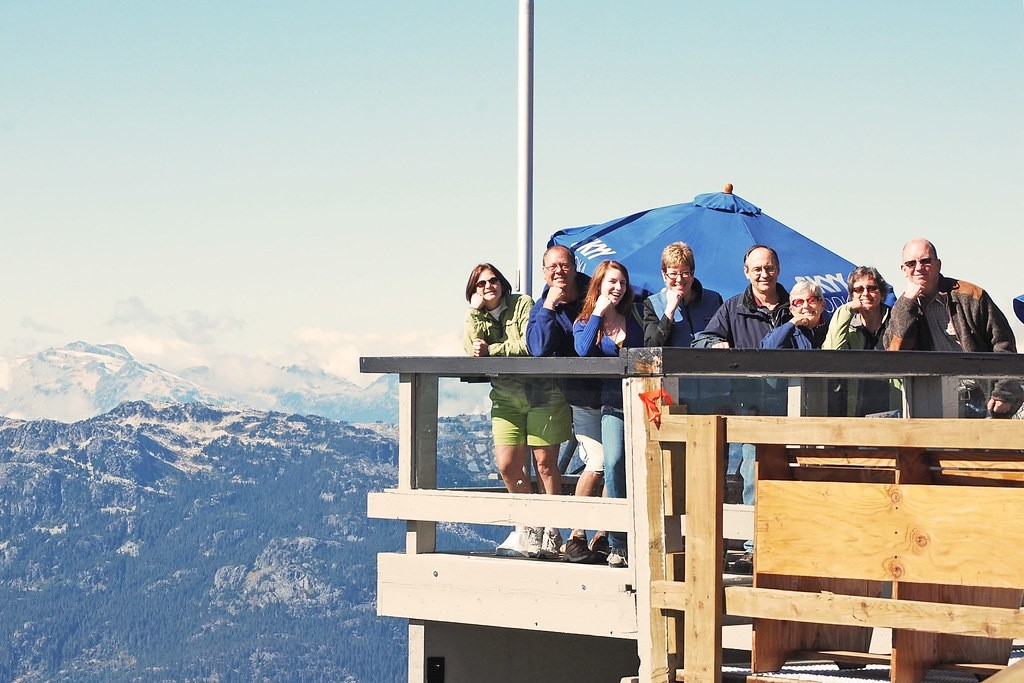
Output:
[821,266,903,417]
[759,280,847,417]
[642,241,727,414]
[526,246,610,562]
[690,245,795,573]
[572,260,646,568]
[880,238,1021,419]
[463,264,573,559]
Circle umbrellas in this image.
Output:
[545,184,898,321]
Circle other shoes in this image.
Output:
[589,534,610,555]
[733,552,752,573]
[563,535,593,563]
[607,551,626,567]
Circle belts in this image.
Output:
[958,388,982,401]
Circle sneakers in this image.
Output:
[543,528,563,559]
[525,526,544,558]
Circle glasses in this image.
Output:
[545,264,574,272]
[851,284,880,293]
[475,277,499,288]
[747,265,777,274]
[791,296,821,308]
[664,271,693,280]
[904,258,935,267]
